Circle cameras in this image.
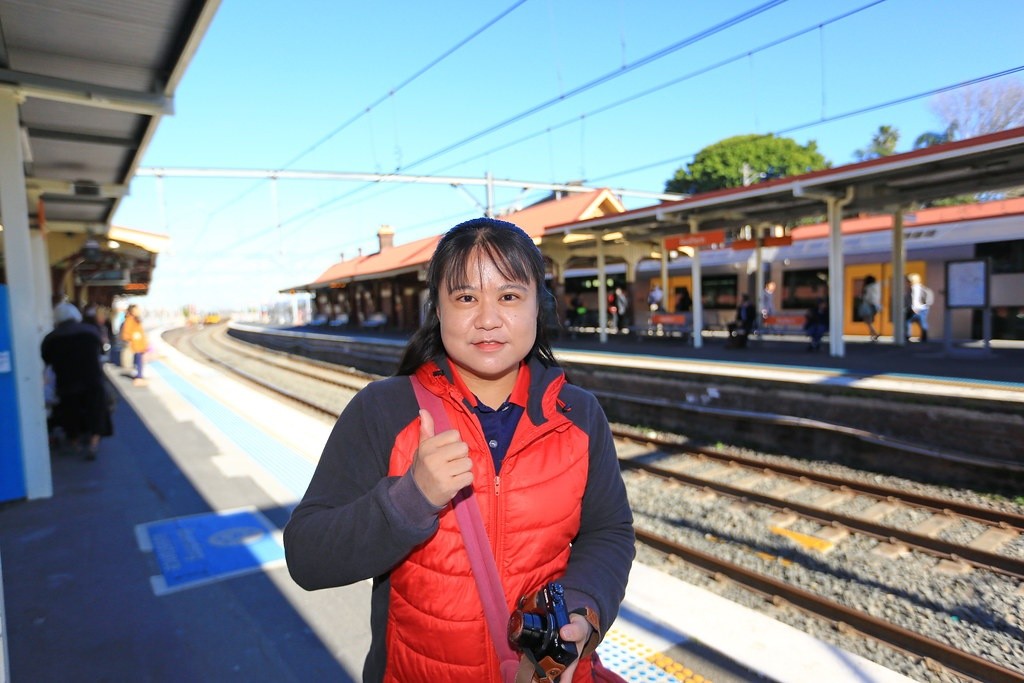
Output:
[508,582,579,683]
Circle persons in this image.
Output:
[41,302,114,462]
[862,276,880,342]
[804,297,830,351]
[563,284,692,341]
[282,217,637,683]
[726,281,777,349]
[904,272,930,342]
[80,302,148,384]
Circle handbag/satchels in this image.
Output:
[120,346,134,368]
[514,607,628,683]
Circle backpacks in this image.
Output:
[922,287,934,306]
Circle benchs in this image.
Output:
[628,309,806,347]
